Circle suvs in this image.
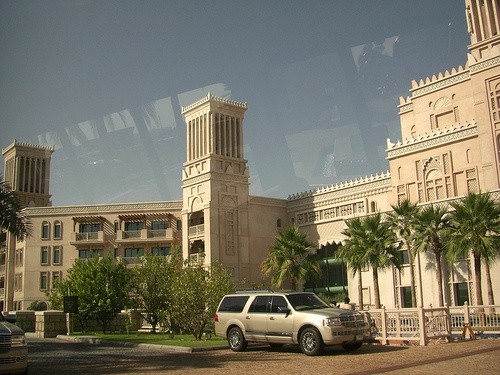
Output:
[0,311,28,374]
[214,288,376,357]
